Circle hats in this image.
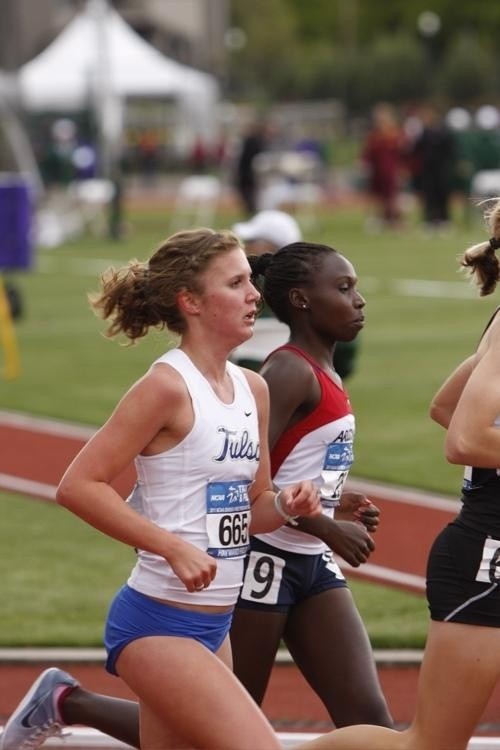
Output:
[232,209,302,250]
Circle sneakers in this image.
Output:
[0,666,80,749]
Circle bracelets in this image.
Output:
[273,489,301,530]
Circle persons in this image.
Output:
[289,197,500,749]
[363,102,462,223]
[226,108,328,378]
[2,242,394,749]
[37,117,228,187]
[51,228,324,749]
